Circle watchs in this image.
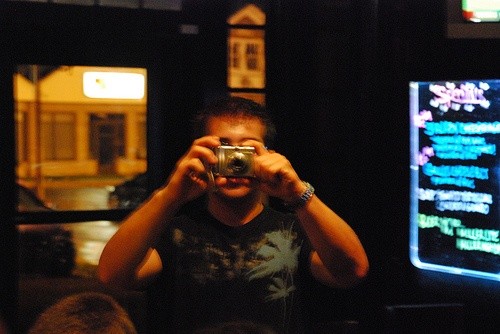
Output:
[284,182,314,211]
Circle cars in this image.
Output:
[17,182,76,278]
[108,173,147,209]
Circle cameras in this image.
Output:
[214,145,257,177]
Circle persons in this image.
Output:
[97,96,370,334]
